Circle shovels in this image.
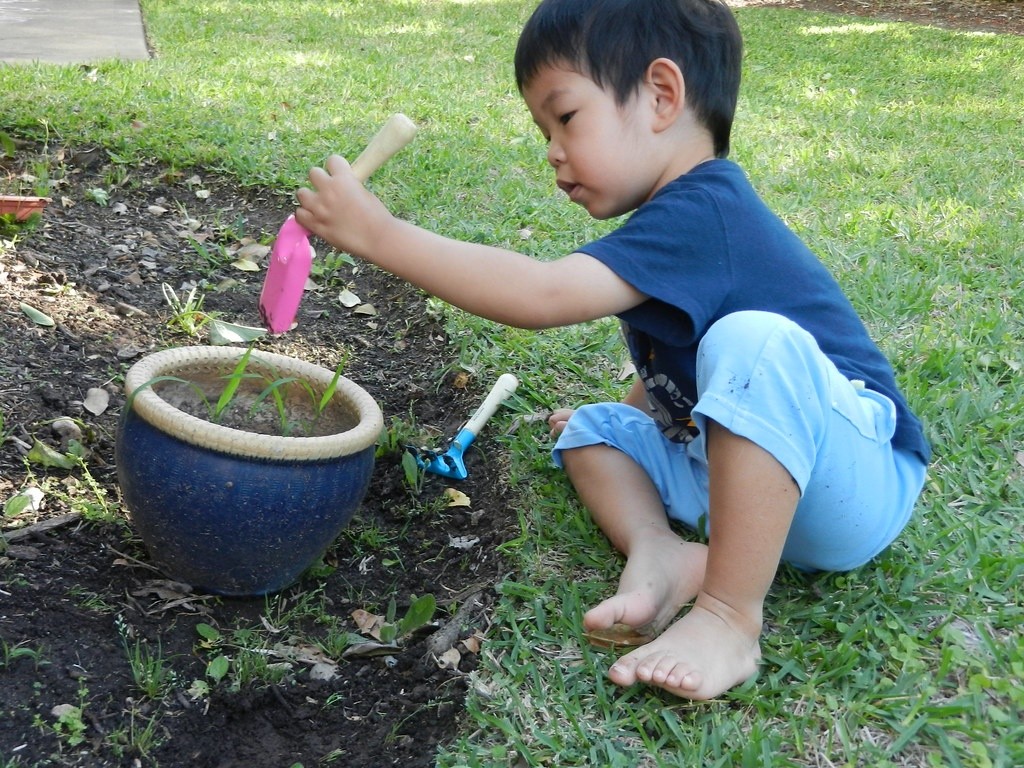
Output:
[255,111,420,337]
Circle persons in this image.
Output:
[297,0,928,700]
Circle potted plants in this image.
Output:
[120,344,382,599]
